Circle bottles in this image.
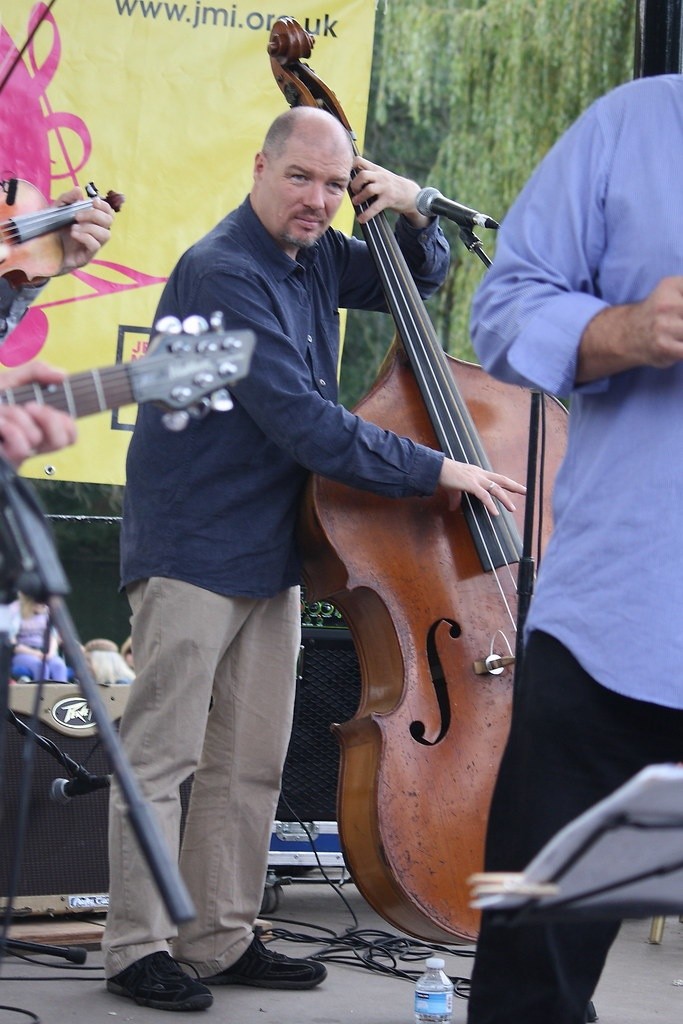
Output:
[414,957,454,1024]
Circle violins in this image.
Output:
[0,177,125,289]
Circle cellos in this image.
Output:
[265,16,571,945]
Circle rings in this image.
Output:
[488,482,495,493]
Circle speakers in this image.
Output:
[0,680,129,918]
[269,626,361,824]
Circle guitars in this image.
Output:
[1,310,257,447]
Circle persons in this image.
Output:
[0,107,529,1015]
[467,72,683,1023]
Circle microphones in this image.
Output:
[48,772,112,804]
[416,186,503,229]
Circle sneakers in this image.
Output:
[195,934,329,990]
[106,951,214,1011]
